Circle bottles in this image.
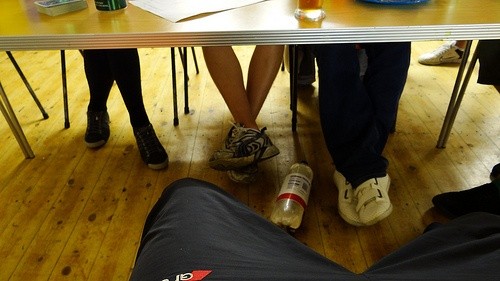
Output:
[271,160,313,229]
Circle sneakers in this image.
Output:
[353,172,392,226]
[418,44,465,65]
[224,123,256,183]
[209,124,280,170]
[84,104,110,147]
[134,125,168,169]
[333,170,367,227]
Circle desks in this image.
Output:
[0,0,500,51]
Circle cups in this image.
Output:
[94,0,128,11]
[295,0,326,23]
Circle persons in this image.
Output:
[129,176,500,281]
[296,39,500,220]
[202,45,285,184]
[83,48,170,170]
